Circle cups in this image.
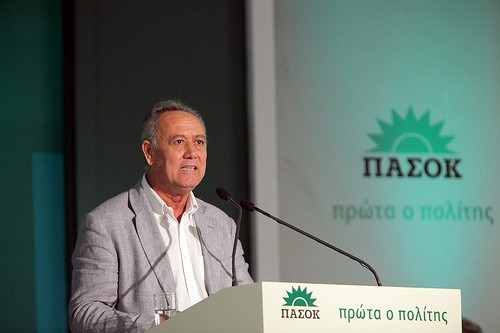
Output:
[153,292,176,326]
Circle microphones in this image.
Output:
[238,199,382,286]
[216,187,243,286]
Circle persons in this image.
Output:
[68,102,255,333]
